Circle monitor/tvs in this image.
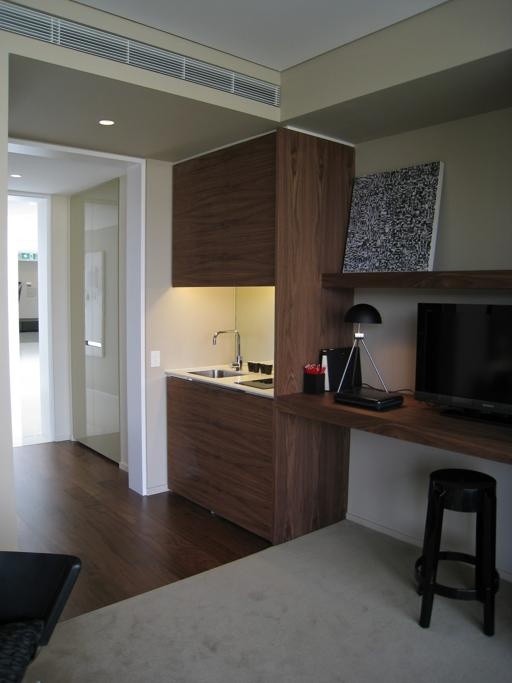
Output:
[414,302,512,428]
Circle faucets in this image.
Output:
[212,329,240,370]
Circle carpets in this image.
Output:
[21,517,512,682]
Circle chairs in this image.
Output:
[0,548,86,681]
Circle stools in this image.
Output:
[410,463,504,637]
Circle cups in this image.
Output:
[247,361,273,375]
[303,373,326,397]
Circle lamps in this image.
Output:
[332,297,393,396]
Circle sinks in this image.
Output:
[189,370,249,379]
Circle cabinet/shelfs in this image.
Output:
[171,129,356,289]
[273,270,511,462]
[163,374,349,544]
